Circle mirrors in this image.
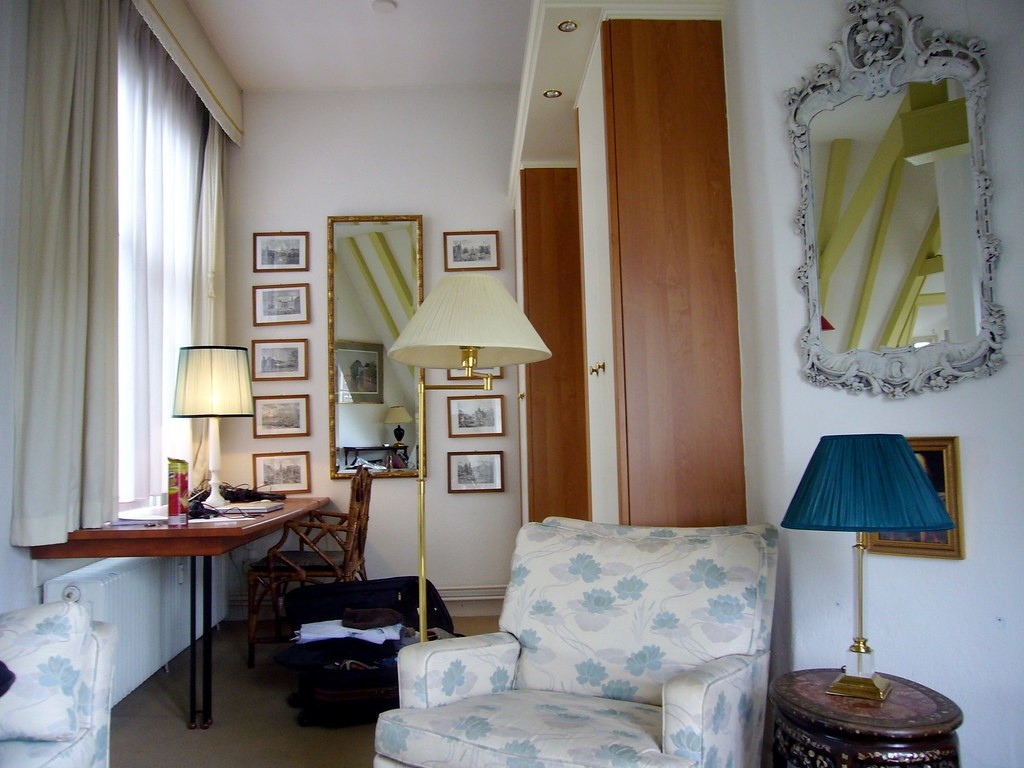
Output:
[780,0,1011,400]
[327,215,427,479]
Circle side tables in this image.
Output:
[762,667,964,768]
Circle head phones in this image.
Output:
[187,500,219,519]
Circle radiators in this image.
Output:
[43,553,229,709]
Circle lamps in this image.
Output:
[387,275,552,391]
[383,405,414,447]
[779,433,957,703]
[172,346,254,508]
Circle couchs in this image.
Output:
[373,516,779,768]
[0,600,117,768]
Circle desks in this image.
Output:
[32,497,329,729]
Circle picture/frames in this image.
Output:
[251,450,312,494]
[252,394,310,439]
[447,395,506,438]
[252,232,310,273]
[447,367,504,380]
[250,338,309,381]
[867,436,966,561]
[251,283,310,327]
[334,340,384,405]
[443,230,501,272]
[447,451,505,493]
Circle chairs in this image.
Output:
[247,467,373,666]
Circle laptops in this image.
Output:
[215,503,284,514]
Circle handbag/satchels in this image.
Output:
[277,577,467,728]
